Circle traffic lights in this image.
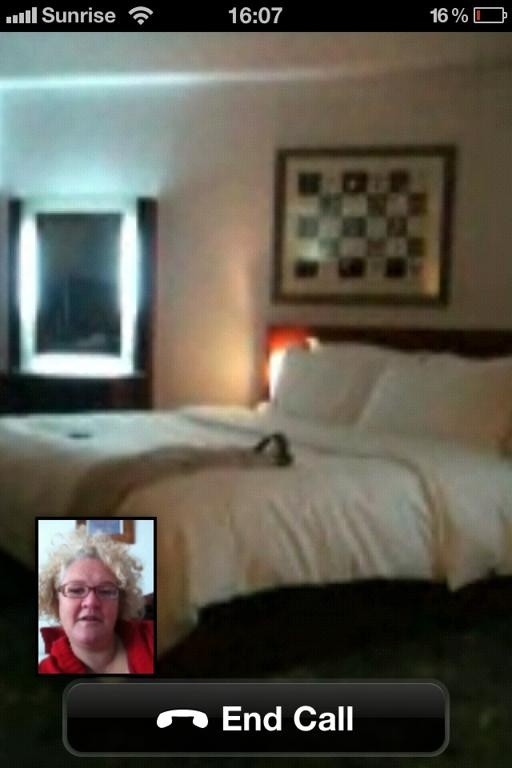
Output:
[274,341,512,455]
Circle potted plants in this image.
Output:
[269,141,456,311]
[71,520,141,545]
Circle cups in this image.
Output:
[55,581,118,600]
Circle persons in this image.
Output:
[38,525,156,679]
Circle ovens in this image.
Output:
[0,320,511,689]
